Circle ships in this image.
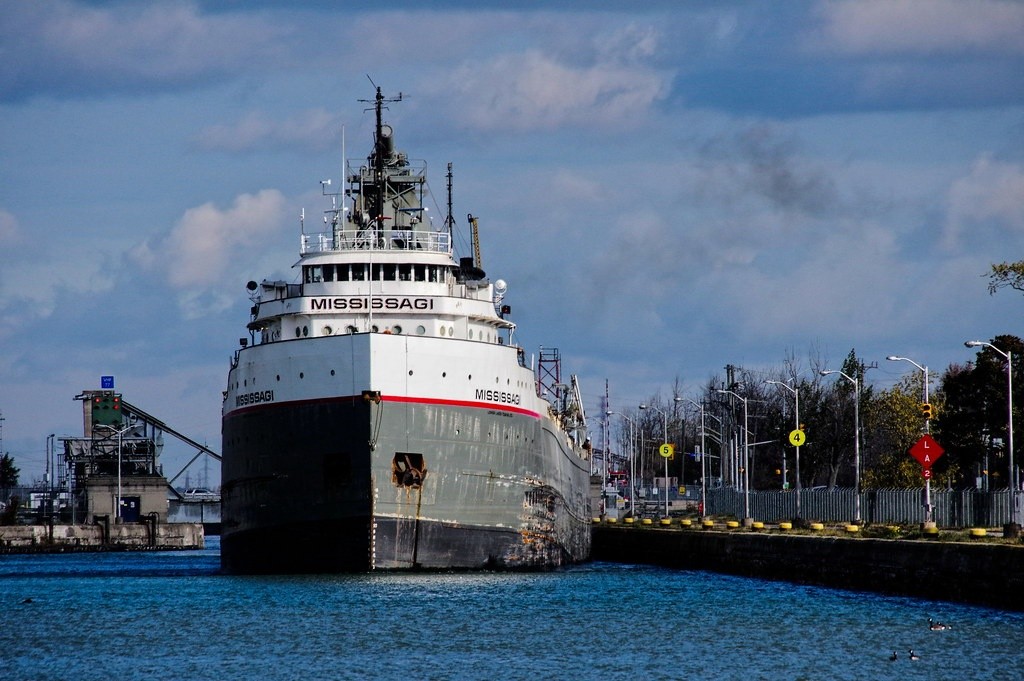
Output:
[217,68,602,577]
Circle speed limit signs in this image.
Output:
[659,444,673,457]
[789,429,806,447]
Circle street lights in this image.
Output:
[763,377,803,518]
[675,397,706,516]
[639,404,669,516]
[715,389,749,520]
[820,368,863,525]
[606,406,635,519]
[963,340,1016,525]
[886,355,931,521]
[96,422,143,517]
